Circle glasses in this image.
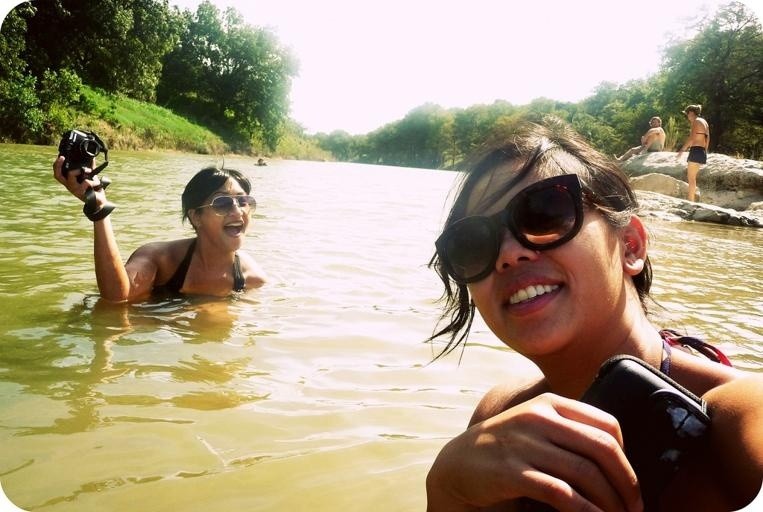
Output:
[434,174,608,282]
[194,195,256,217]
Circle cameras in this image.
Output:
[58,129,101,170]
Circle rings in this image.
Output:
[66,184,72,190]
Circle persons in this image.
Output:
[254,159,268,166]
[424,113,763,512]
[612,116,666,165]
[53,154,268,303]
[675,104,711,202]
